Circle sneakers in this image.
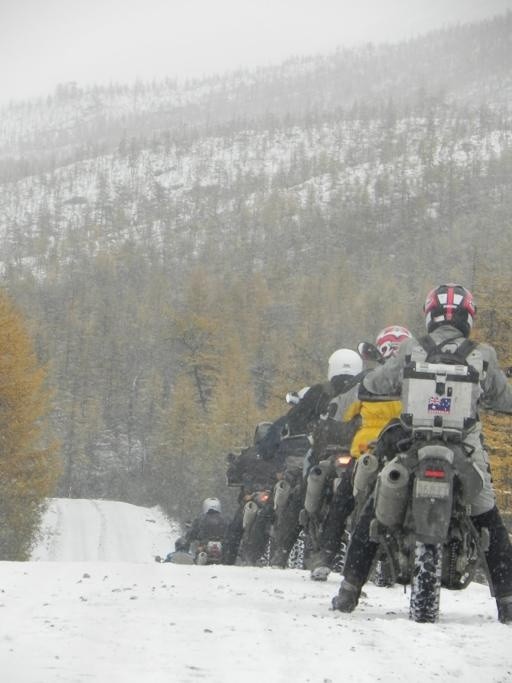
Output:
[332,582,361,614]
[310,565,331,582]
[496,591,512,625]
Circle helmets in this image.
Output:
[202,498,223,515]
[326,348,362,388]
[377,325,413,360]
[298,387,311,400]
[422,283,477,338]
[253,422,273,446]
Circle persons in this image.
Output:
[221,347,366,580]
[165,497,227,564]
[331,286,511,623]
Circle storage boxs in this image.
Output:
[402,361,477,430]
